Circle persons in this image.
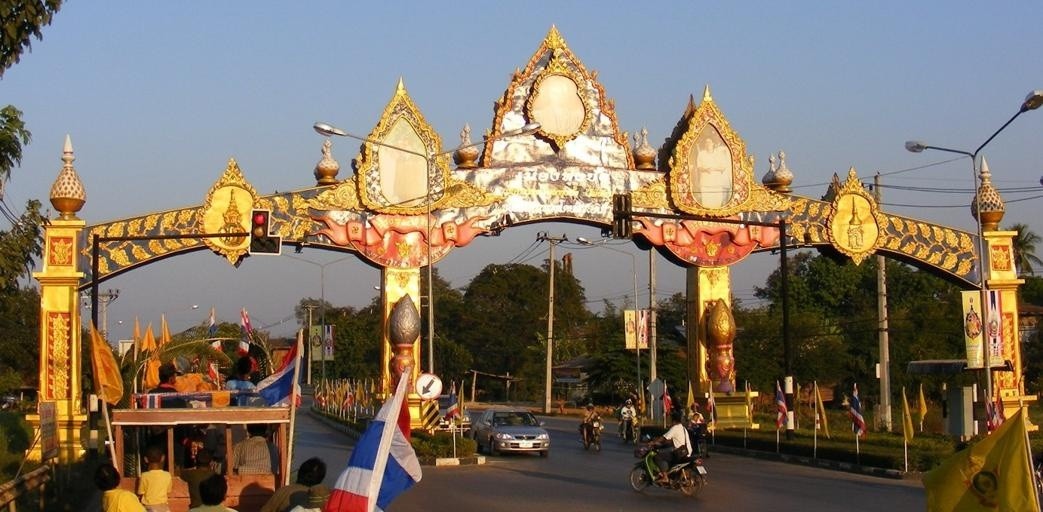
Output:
[181,450,216,510]
[230,423,279,475]
[620,398,636,441]
[146,364,188,409]
[259,457,330,512]
[93,462,147,511]
[696,134,726,209]
[648,412,693,483]
[582,402,605,438]
[135,443,174,511]
[687,403,710,457]
[187,473,239,511]
[123,423,225,475]
[224,355,257,407]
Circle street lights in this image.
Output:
[576,235,644,411]
[298,303,324,385]
[905,88,1042,430]
[102,322,128,340]
[372,285,381,294]
[159,304,202,345]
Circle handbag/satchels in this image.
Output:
[672,445,688,459]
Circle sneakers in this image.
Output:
[655,479,669,483]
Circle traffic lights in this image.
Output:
[248,210,286,258]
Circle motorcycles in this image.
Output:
[616,411,644,447]
[577,415,609,453]
[680,420,715,458]
[628,434,710,497]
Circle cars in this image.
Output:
[2,386,40,411]
[437,394,473,436]
[471,407,552,457]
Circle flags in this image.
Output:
[983,387,998,432]
[901,388,914,444]
[133,319,140,341]
[90,323,123,406]
[919,408,1038,511]
[208,310,217,338]
[192,341,221,382]
[253,344,304,409]
[312,377,375,410]
[158,317,172,346]
[442,386,460,424]
[664,384,672,417]
[744,391,752,428]
[239,310,252,335]
[919,388,927,423]
[141,324,156,355]
[686,382,694,408]
[705,385,716,413]
[849,388,866,438]
[815,384,830,440]
[774,383,789,430]
[454,383,463,426]
[327,372,422,512]
[995,390,1006,426]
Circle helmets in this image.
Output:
[625,400,632,408]
